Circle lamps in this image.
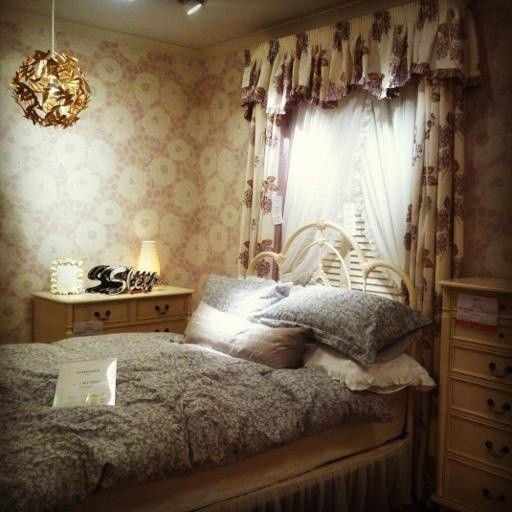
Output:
[136,240,160,277]
[178,1,206,16]
[6,0,90,130]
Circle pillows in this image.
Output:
[182,273,437,393]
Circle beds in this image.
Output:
[1,222,417,511]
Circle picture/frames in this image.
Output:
[49,258,86,297]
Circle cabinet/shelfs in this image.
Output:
[429,275,512,511]
[30,280,196,343]
